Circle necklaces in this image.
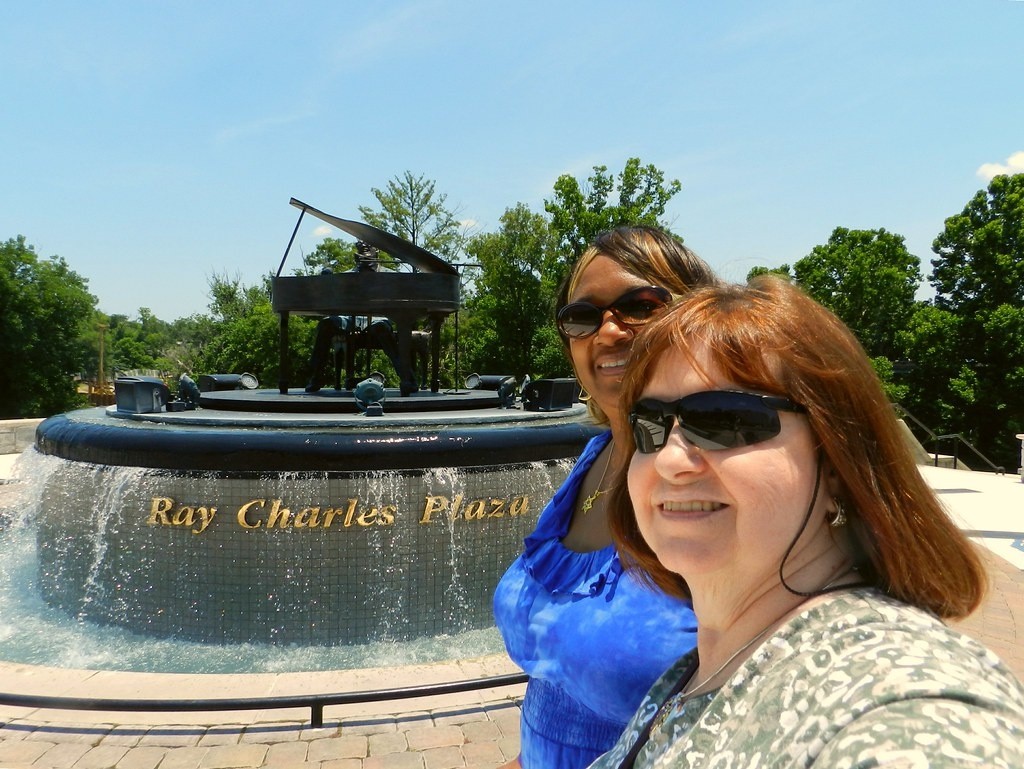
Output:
[647,564,858,732]
[581,441,618,513]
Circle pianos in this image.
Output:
[269,196,462,397]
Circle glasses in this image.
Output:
[557,286,686,340]
[627,389,809,454]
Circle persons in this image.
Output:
[304,236,418,396]
[492,227,719,769]
[584,274,1023,769]
